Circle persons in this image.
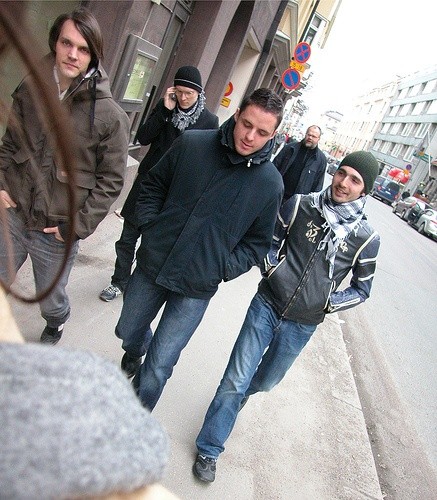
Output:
[115,86,289,416]
[0,2,179,498]
[99,67,328,311]
[400,188,411,198]
[193,150,384,483]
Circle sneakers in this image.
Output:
[238,395,249,413]
[193,453,217,482]
[40,322,64,346]
[121,351,142,379]
[99,285,124,302]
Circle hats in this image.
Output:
[173,66,203,93]
[338,151,379,195]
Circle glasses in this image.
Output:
[176,90,196,95]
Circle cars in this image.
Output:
[322,151,343,176]
[392,196,437,241]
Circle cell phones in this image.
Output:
[171,92,175,98]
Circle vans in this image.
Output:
[370,175,405,206]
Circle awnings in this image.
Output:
[388,167,410,182]
